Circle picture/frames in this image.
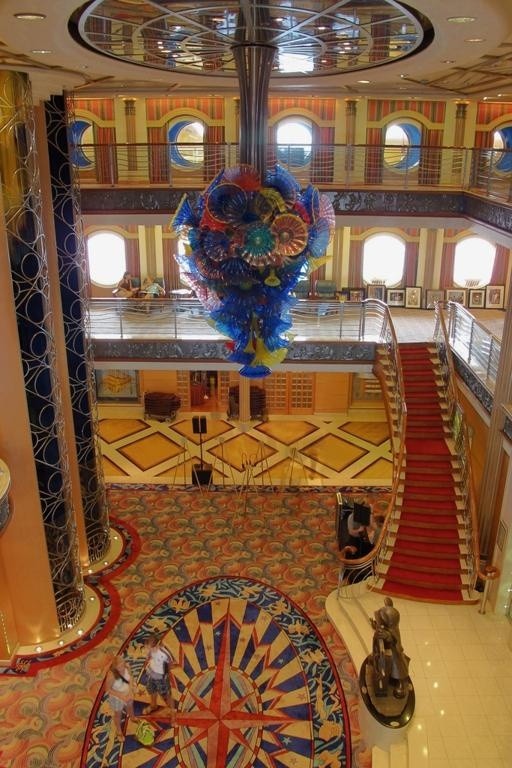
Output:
[334,284,504,310]
[95,369,140,402]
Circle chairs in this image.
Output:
[112,278,165,317]
[291,280,338,315]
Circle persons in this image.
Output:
[372,515,388,552]
[144,277,166,299]
[347,511,367,541]
[115,272,133,298]
